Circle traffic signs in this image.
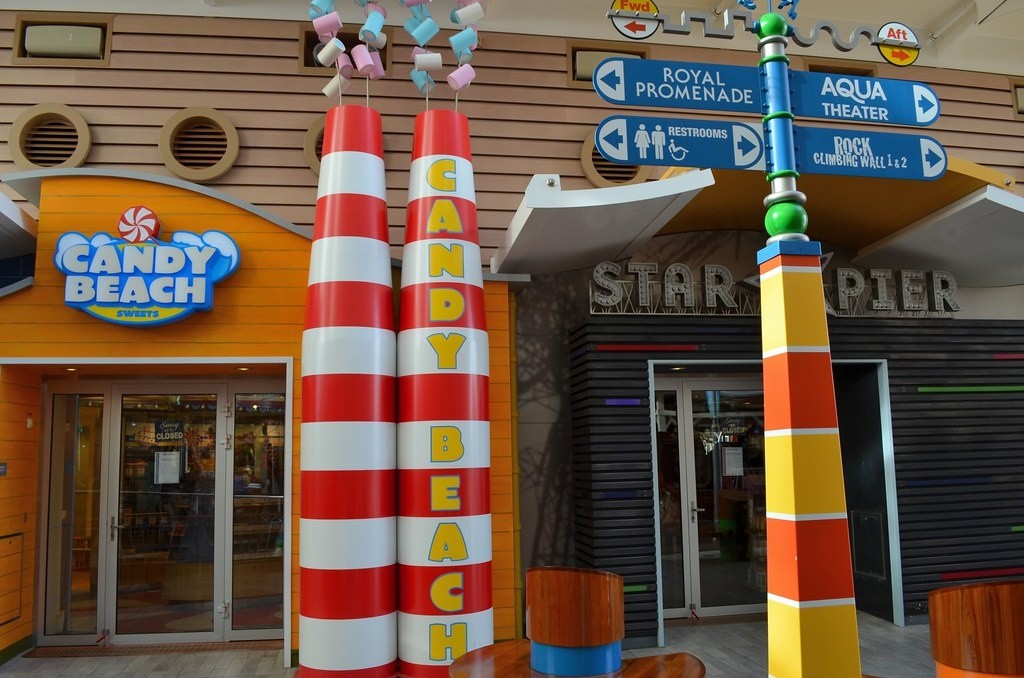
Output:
[592,56,947,179]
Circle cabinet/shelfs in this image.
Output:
[90,445,284,602]
[744,474,767,595]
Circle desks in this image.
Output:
[449,566,706,678]
